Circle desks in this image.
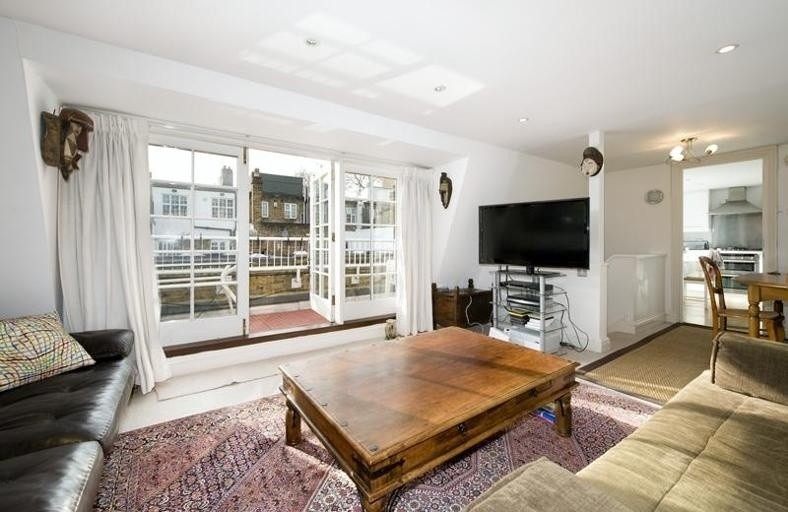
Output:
[733,273,788,342]
[431,278,494,330]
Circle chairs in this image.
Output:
[195,263,236,318]
[698,256,780,342]
[683,251,719,309]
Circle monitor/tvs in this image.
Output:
[478,197,589,275]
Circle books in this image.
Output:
[508,308,554,331]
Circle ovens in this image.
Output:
[717,254,760,294]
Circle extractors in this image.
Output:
[708,186,763,216]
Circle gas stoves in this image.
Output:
[718,247,762,254]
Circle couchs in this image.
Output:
[462,330,788,512]
[0,329,137,512]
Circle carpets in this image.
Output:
[154,336,386,402]
[92,378,660,512]
[575,320,768,404]
[249,308,330,334]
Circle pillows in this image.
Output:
[0,311,96,392]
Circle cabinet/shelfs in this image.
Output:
[489,270,567,355]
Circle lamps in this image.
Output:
[665,135,719,164]
